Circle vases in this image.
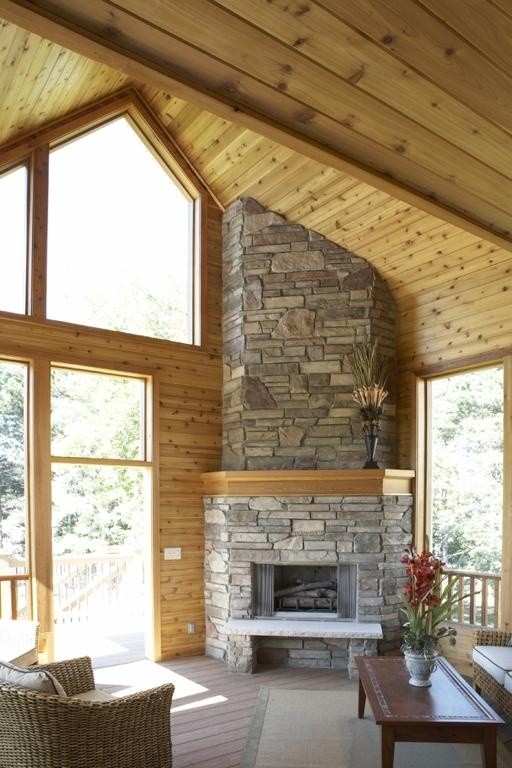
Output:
[404,649,438,688]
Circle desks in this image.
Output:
[353,656,505,768]
[222,620,384,673]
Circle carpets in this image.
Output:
[239,687,511,767]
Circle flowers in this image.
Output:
[398,535,481,652]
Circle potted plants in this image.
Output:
[344,336,394,468]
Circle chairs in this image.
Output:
[0,656,174,768]
[473,629,512,720]
[0,618,39,668]
